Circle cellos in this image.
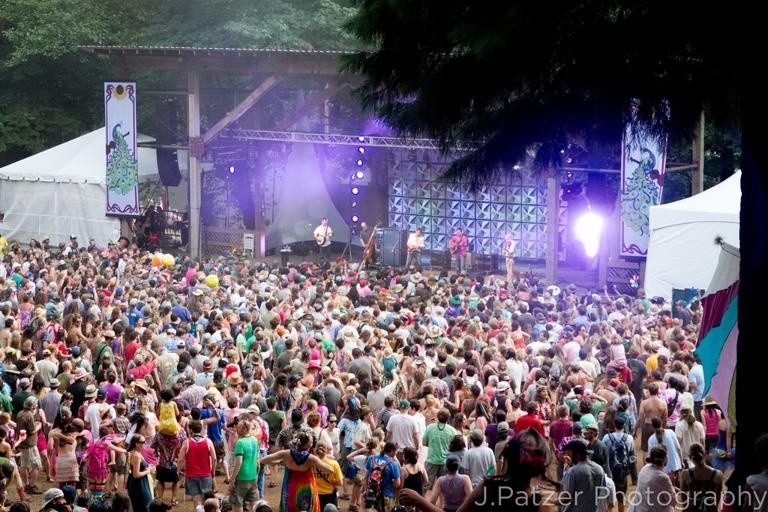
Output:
[362,223,384,259]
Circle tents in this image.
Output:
[0,127,215,251]
[643,169,743,299]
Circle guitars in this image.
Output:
[449,239,469,253]
[408,245,426,254]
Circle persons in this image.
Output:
[0,206,742,512]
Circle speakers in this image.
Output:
[375,228,407,266]
[431,249,451,271]
[156,106,182,186]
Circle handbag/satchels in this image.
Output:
[156,465,179,482]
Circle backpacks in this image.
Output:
[609,432,630,477]
[367,466,384,496]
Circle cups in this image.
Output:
[20,430,26,436]
[36,422,40,427]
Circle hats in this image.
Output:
[4,329,148,432]
[244,405,260,415]
[472,428,484,441]
[41,488,64,510]
[581,425,599,434]
[503,428,552,469]
[192,290,202,296]
[451,295,461,305]
[277,322,340,388]
[410,274,422,283]
[700,395,717,406]
[497,421,510,432]
[495,381,509,393]
[168,326,264,404]
